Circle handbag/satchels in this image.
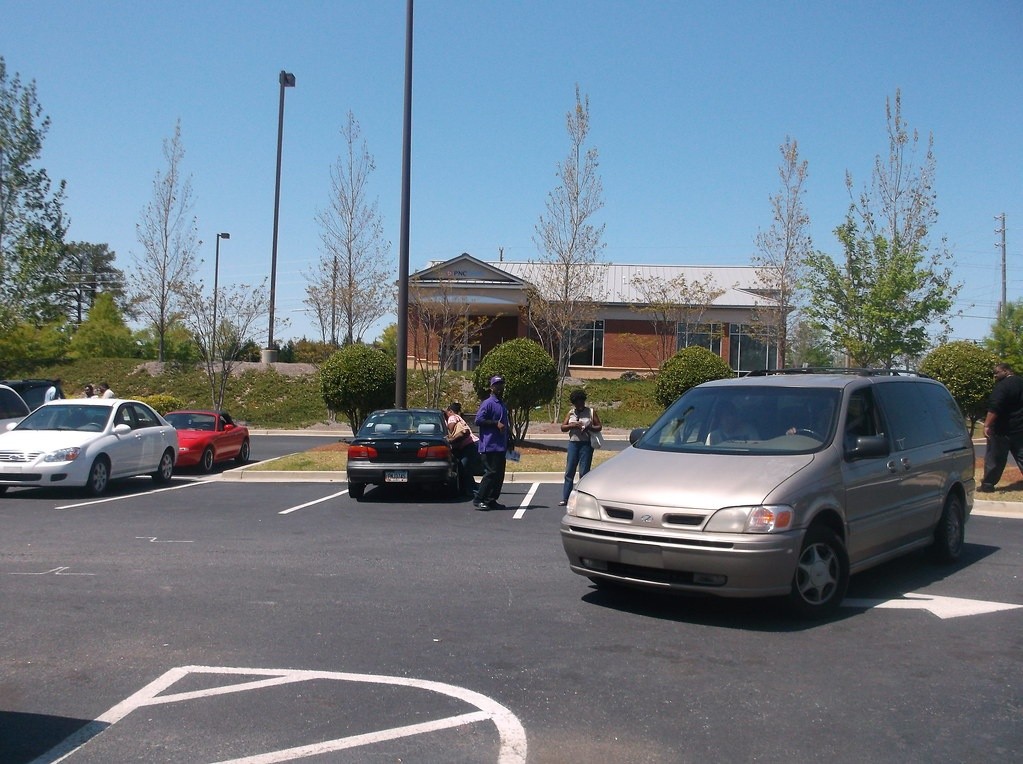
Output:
[446,415,470,444]
[589,408,604,449]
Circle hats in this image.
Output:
[449,403,461,414]
[490,376,503,385]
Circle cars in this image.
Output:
[343,408,462,501]
[0,385,30,430]
[0,396,179,496]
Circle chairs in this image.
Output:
[65,410,87,428]
[375,423,392,432]
[93,415,105,425]
[418,424,435,435]
[174,416,186,426]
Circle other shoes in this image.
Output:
[558,501,568,506]
[489,500,506,509]
[977,486,994,492]
[472,501,490,510]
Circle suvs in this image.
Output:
[559,367,978,623]
[2,378,67,412]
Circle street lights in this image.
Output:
[258,71,296,363]
[211,233,233,360]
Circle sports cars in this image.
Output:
[160,409,250,475]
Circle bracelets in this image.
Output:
[984,426,989,427]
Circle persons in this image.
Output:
[785,398,823,436]
[976,363,1023,493]
[44,379,61,404]
[705,401,763,447]
[473,376,514,511]
[98,382,115,398]
[558,390,602,505]
[444,403,483,499]
[84,385,99,399]
